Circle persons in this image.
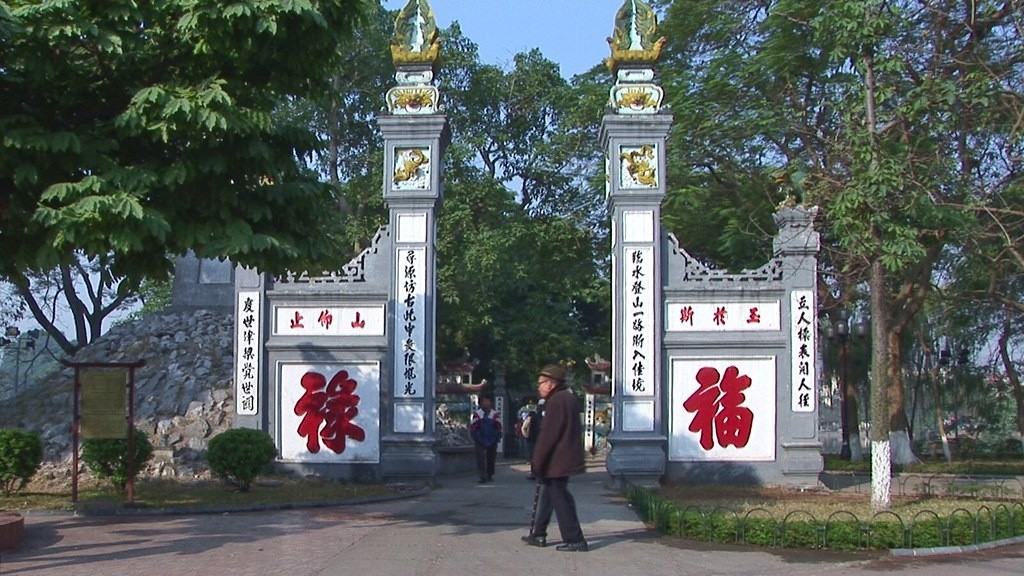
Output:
[517,397,589,465]
[520,364,589,552]
[470,393,504,485]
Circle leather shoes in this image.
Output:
[521,536,546,547]
[557,540,588,552]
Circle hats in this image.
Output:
[536,363,567,382]
[538,399,546,405]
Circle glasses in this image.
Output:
[536,379,549,388]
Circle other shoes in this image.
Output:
[479,477,488,483]
[488,473,492,482]
